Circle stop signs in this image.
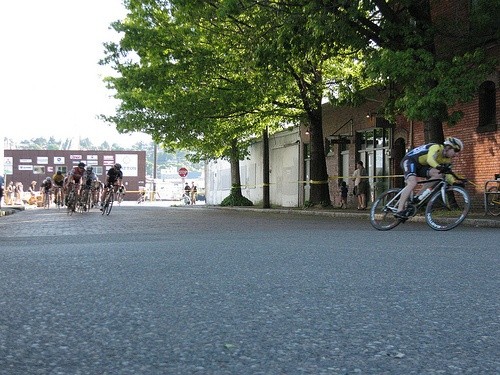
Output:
[178,168,188,177]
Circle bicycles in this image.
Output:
[40,177,125,215]
[369,163,471,232]
[484,173,500,217]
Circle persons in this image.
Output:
[138,188,146,203]
[351,160,368,209]
[41,162,126,213]
[0,175,37,210]
[396,136,466,214]
[337,181,348,209]
[182,182,197,205]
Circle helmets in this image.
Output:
[87,167,93,171]
[443,137,463,152]
[57,171,61,175]
[113,163,121,173]
[46,177,51,181]
[78,163,85,166]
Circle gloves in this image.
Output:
[437,163,451,171]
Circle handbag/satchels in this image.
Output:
[353,186,357,195]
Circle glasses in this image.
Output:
[451,148,460,153]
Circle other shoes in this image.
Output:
[100,204,103,211]
[394,211,409,220]
